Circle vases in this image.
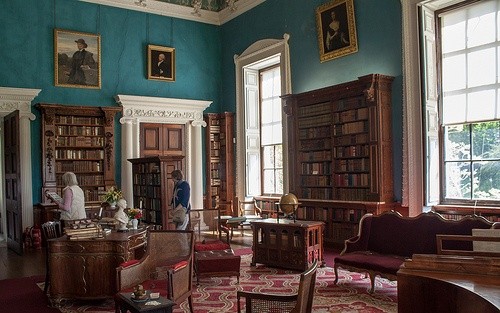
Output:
[132,219,138,229]
[108,201,116,207]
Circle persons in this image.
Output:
[113,199,129,232]
[170,170,191,230]
[53,171,87,228]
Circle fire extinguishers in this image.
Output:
[23,227,30,250]
[29,224,41,250]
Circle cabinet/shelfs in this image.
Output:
[33,102,122,202]
[50,201,118,222]
[128,124,186,229]
[281,73,394,202]
[250,216,326,271]
[396,263,500,313]
[255,197,394,245]
[204,111,237,217]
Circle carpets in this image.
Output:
[0,262,398,313]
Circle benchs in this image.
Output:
[333,210,500,293]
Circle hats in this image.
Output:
[74,39,87,48]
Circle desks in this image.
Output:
[116,289,177,313]
[46,229,148,300]
[194,250,240,286]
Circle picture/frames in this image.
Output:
[54,28,102,89]
[315,0,358,64]
[146,45,176,82]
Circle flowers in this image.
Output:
[125,208,143,219]
[101,186,122,202]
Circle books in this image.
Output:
[210,125,223,210]
[297,95,372,245]
[56,116,113,238]
[134,162,163,230]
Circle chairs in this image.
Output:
[237,258,318,313]
[115,230,194,313]
[212,200,261,242]
[42,219,63,297]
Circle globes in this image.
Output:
[275,193,302,223]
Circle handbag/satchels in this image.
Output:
[172,206,187,225]
